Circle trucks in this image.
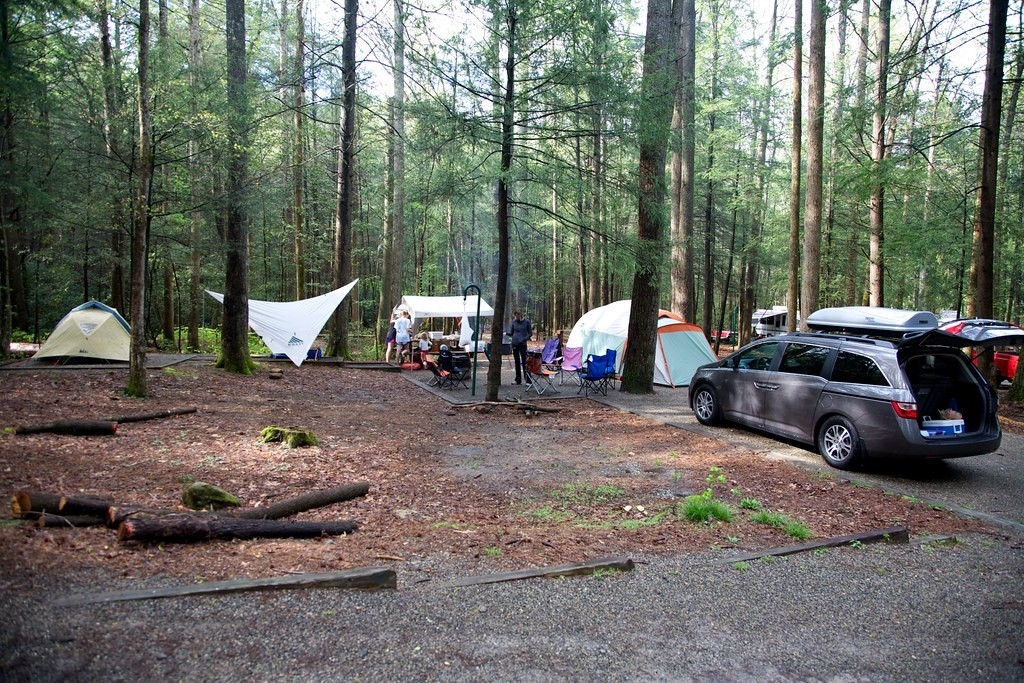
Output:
[748,307,808,337]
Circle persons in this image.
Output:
[430,344,454,387]
[418,331,432,364]
[502,308,533,387]
[393,309,413,365]
[385,315,400,367]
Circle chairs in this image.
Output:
[587,348,619,390]
[438,351,471,391]
[524,351,559,396]
[555,346,584,387]
[572,352,608,397]
[423,359,453,387]
[519,337,560,376]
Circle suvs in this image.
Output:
[688,306,1024,468]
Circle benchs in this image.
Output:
[401,351,440,364]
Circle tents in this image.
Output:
[390,293,496,372]
[30,297,133,362]
[561,298,722,390]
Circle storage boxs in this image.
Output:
[921,414,965,435]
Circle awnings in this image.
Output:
[750,309,788,339]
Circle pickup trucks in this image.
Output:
[942,319,1024,386]
